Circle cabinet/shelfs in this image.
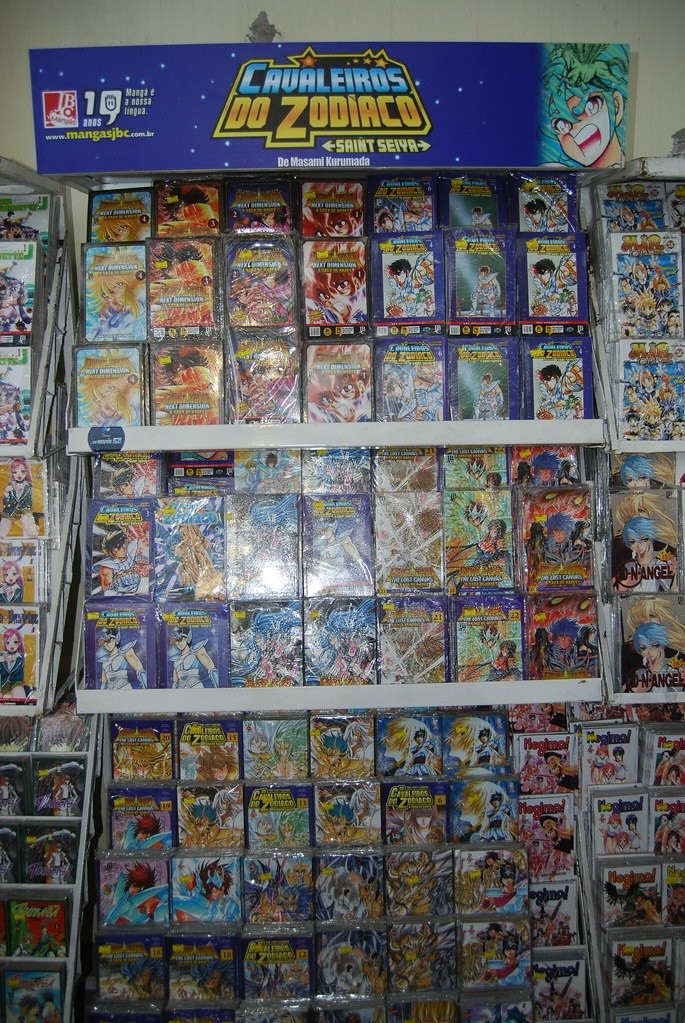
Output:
[0,158,685,1023]
